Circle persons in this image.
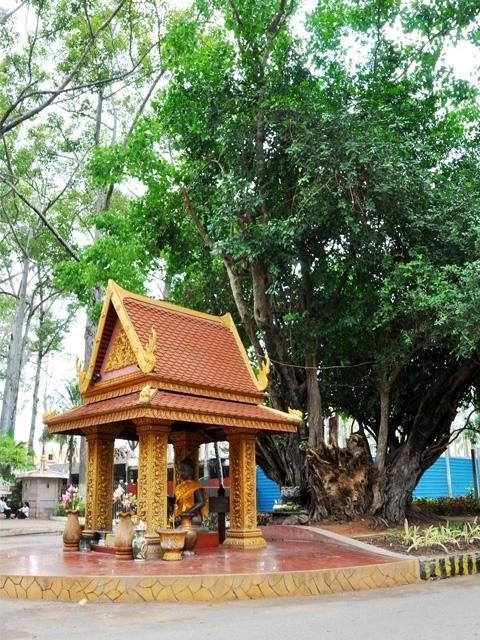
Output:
[11,501,30,519]
[0,496,5,505]
[0,497,12,519]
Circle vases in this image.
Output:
[63,509,81,552]
[131,529,148,562]
[114,511,135,561]
[176,514,199,557]
[155,530,187,561]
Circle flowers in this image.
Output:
[112,481,138,512]
[135,520,148,530]
[61,485,82,510]
